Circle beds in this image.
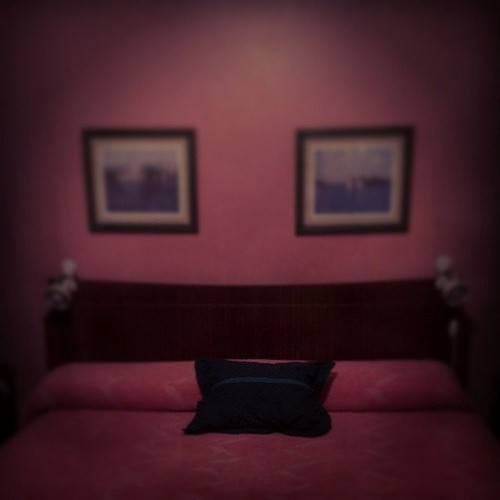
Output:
[0,277,499,500]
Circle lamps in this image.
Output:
[434,256,470,306]
[46,259,77,309]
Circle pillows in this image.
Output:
[183,357,336,437]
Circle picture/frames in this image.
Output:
[82,126,198,233]
[294,125,413,236]
